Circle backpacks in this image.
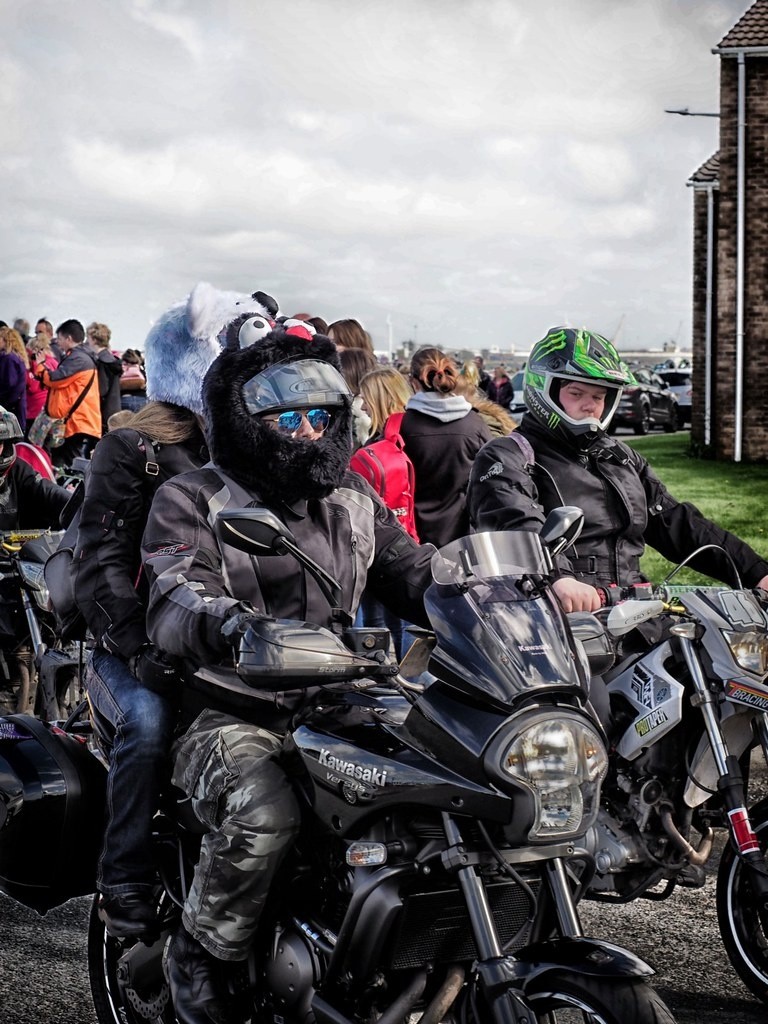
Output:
[44,428,160,641]
[349,414,419,547]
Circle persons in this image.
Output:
[296,305,513,663]
[1,313,147,526]
[140,315,482,1023]
[83,283,281,939]
[468,324,768,882]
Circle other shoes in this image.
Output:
[665,865,706,889]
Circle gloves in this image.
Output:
[221,612,273,652]
[132,649,184,696]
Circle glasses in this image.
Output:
[263,408,332,435]
[217,335,228,347]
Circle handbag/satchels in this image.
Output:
[27,405,67,449]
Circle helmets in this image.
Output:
[521,327,637,457]
[242,354,354,443]
[0,405,24,470]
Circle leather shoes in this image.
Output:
[161,926,229,1024]
[97,890,155,935]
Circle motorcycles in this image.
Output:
[0,457,768,1024]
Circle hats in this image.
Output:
[144,283,279,417]
[201,313,354,501]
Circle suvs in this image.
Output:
[651,369,692,430]
[510,368,679,435]
